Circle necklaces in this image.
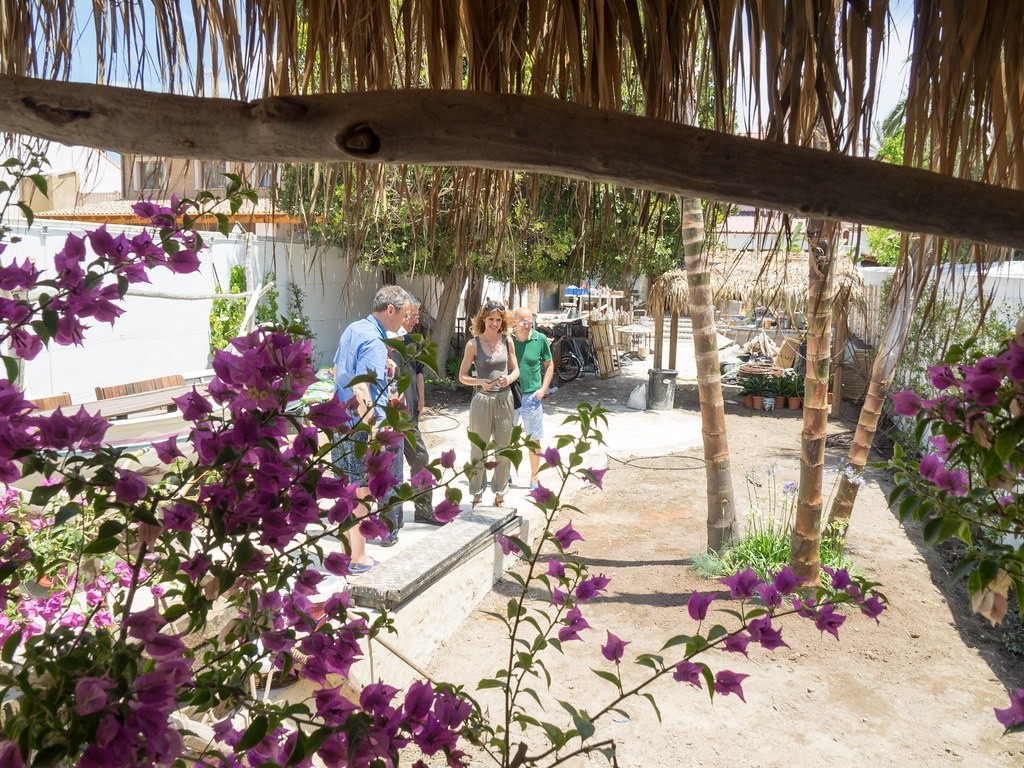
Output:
[483,333,498,352]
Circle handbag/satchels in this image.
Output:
[508,369,523,409]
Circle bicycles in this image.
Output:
[553,352,583,381]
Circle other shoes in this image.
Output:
[348,556,381,575]
[529,481,539,491]
[381,537,398,547]
[495,498,505,507]
[414,511,449,526]
[472,500,482,510]
[486,476,512,486]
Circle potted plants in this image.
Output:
[737,375,805,412]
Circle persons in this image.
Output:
[458,301,520,507]
[509,308,554,490]
[331,284,453,573]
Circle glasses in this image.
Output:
[410,315,420,320]
[485,303,505,312]
[519,320,533,326]
[401,310,408,319]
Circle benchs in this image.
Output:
[29,386,211,421]
